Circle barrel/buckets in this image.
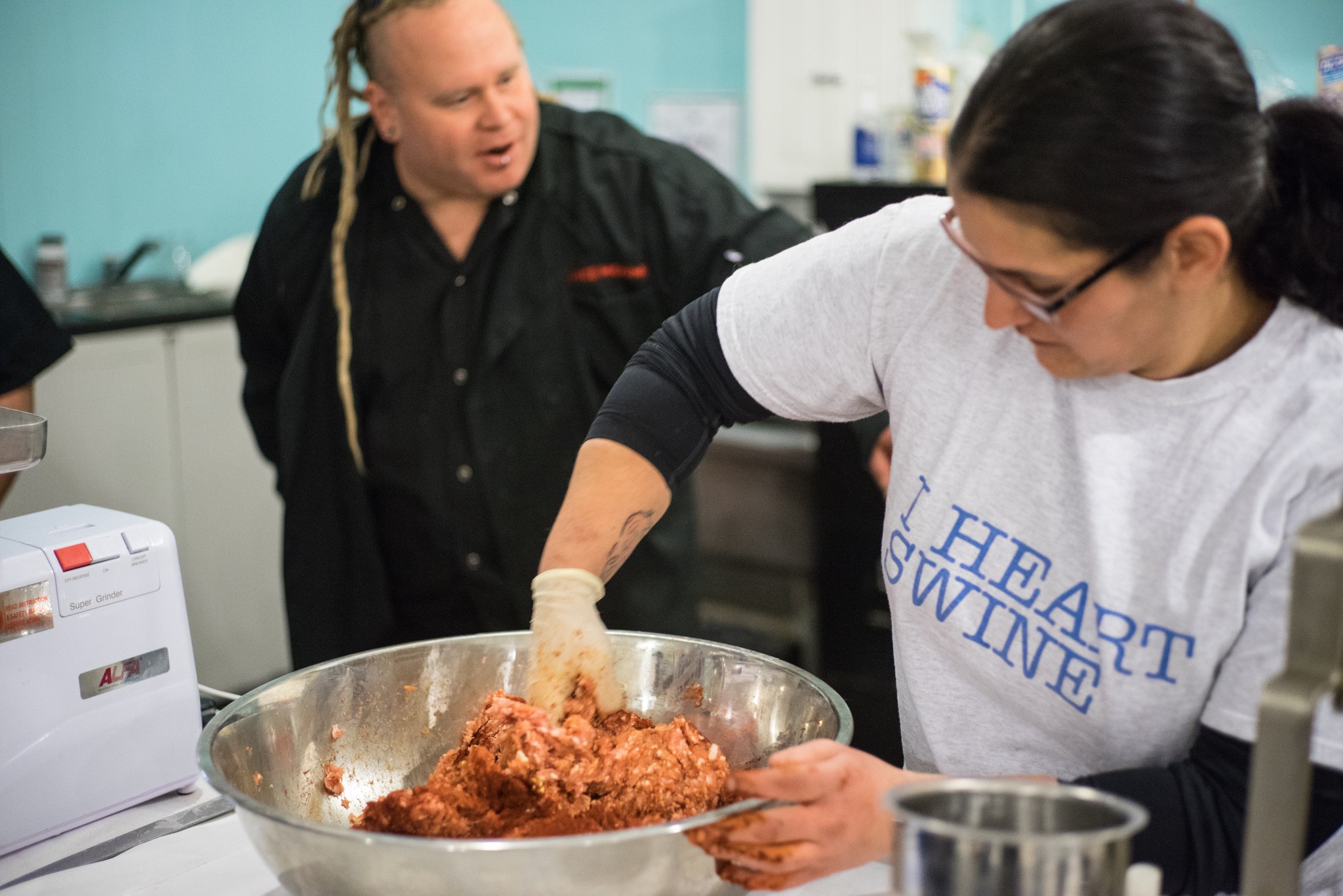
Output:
[881,774,1149,895]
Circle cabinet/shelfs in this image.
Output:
[17,296,307,708]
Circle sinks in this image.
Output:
[49,280,199,309]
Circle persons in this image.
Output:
[0,239,76,508]
[232,0,894,673]
[522,0,1343,896]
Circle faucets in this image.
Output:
[104,241,160,286]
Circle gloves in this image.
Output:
[524,568,626,724]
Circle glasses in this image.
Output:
[941,205,1171,322]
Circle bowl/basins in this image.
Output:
[198,620,854,890]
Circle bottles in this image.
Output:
[852,86,891,184]
[38,228,68,305]
[906,55,952,187]
